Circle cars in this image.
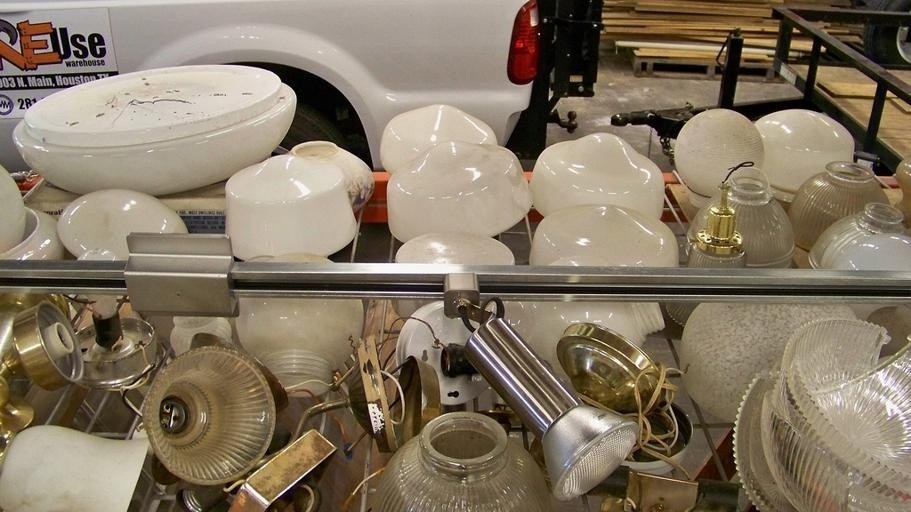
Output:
[0,2,610,176]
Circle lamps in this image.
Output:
[443,274,641,504]
